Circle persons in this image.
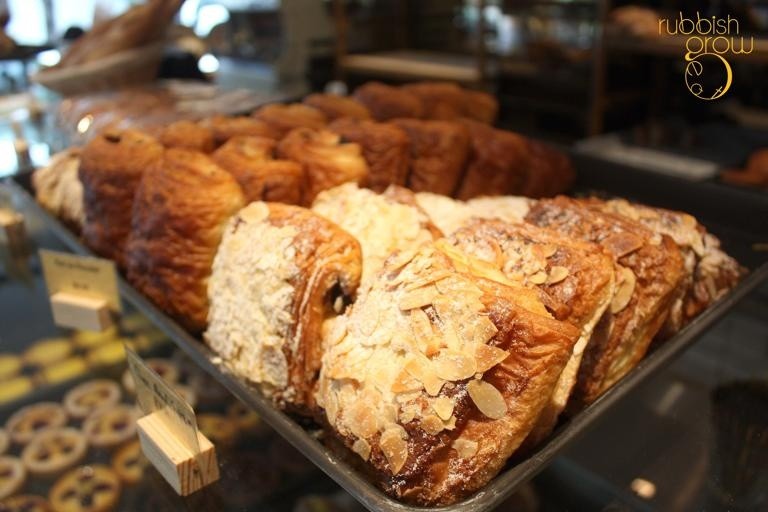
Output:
[602,0,767,138]
[0,0,53,57]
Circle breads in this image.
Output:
[30,2,749,508]
